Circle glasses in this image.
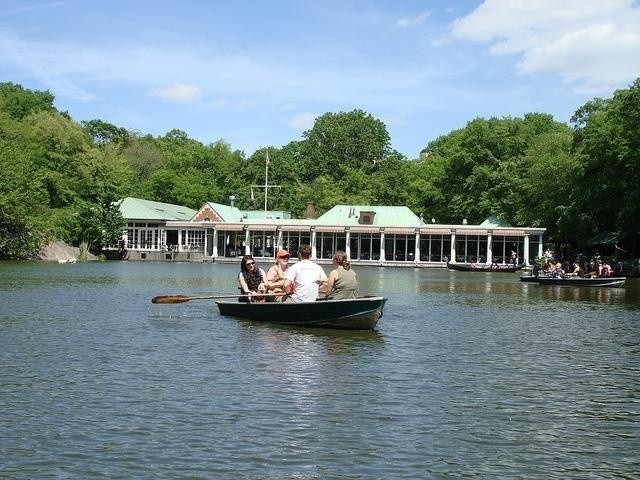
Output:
[246,262,254,266]
[279,256,289,259]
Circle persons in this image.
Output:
[491,262,499,270]
[314,250,360,301]
[275,245,327,303]
[533,248,625,279]
[470,261,477,268]
[238,256,267,302]
[264,249,290,302]
[510,250,518,269]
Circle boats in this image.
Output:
[446,260,526,274]
[214,294,389,330]
[519,275,628,289]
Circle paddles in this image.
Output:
[152,293,288,304]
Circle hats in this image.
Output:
[277,249,291,258]
